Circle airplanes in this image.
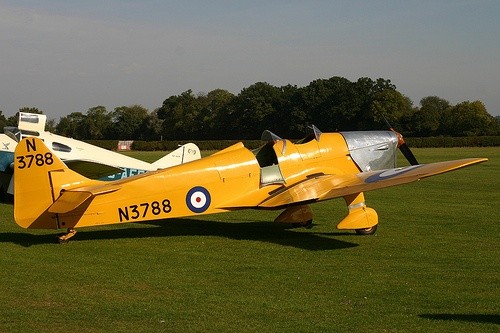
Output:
[14,101,488,245]
[0,111,201,202]
